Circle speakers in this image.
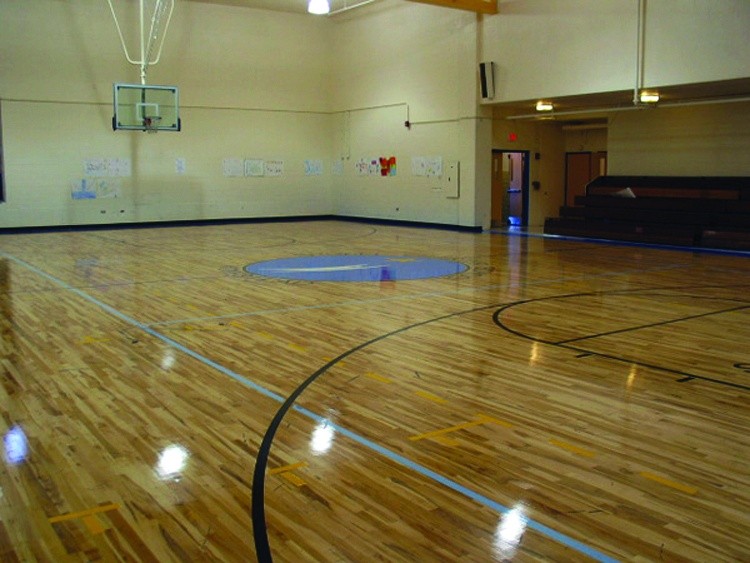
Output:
[480,61,494,98]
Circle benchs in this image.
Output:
[544,176,750,251]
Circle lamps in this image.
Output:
[640,89,660,103]
[536,99,553,112]
